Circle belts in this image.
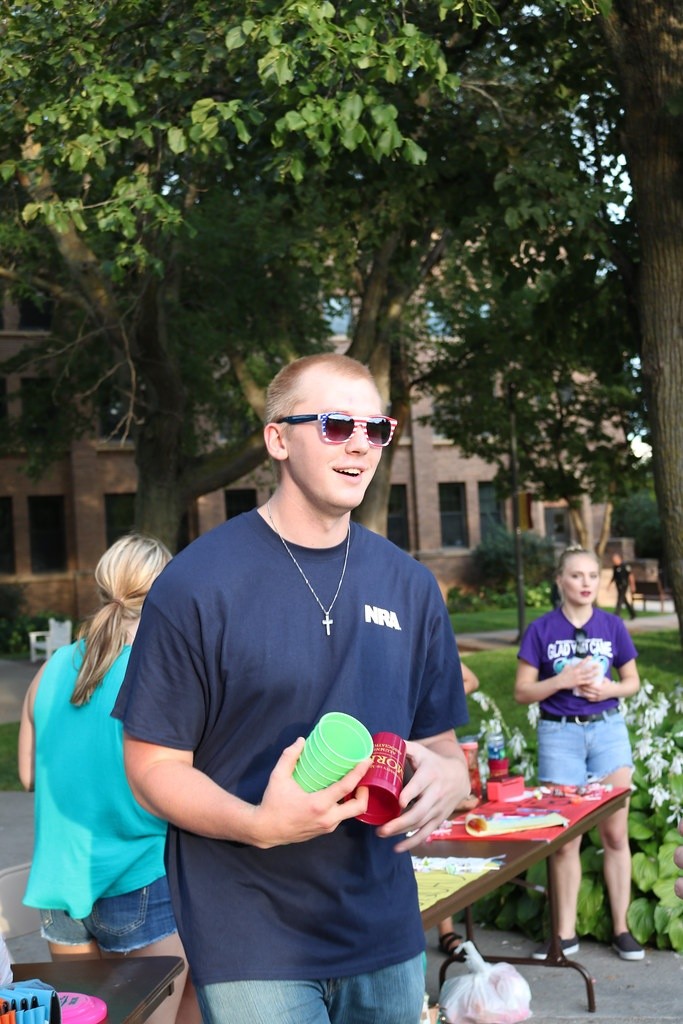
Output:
[540,704,622,723]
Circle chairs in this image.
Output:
[29,617,72,662]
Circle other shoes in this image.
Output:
[531,937,579,960]
[611,932,645,959]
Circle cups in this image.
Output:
[343,731,406,825]
[293,712,374,793]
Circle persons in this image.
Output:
[513,542,642,962]
[673,814,683,905]
[17,533,190,1024]
[111,351,481,1024]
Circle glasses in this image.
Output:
[275,411,398,447]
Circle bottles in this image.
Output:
[484,718,505,760]
[456,734,481,802]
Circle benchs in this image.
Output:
[631,580,671,612]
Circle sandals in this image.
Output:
[437,933,469,961]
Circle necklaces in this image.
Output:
[267,499,352,637]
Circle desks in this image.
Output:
[410,786,633,1012]
[11,955,186,1024]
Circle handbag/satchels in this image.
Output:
[437,941,533,1024]
